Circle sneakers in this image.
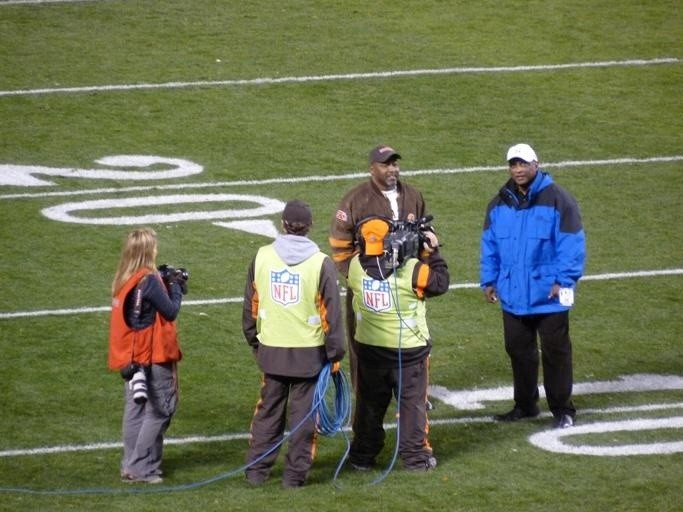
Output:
[121,475,163,483]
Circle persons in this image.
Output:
[328,146,433,411]
[349,214,450,472]
[108,227,183,485]
[241,200,348,489]
[479,144,587,429]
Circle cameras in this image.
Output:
[156,263,188,283]
[120,362,149,404]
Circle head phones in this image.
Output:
[351,214,393,246]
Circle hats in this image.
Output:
[360,219,389,256]
[369,146,402,166]
[281,201,311,227]
[507,144,537,164]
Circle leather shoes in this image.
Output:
[495,407,540,421]
[552,414,576,429]
